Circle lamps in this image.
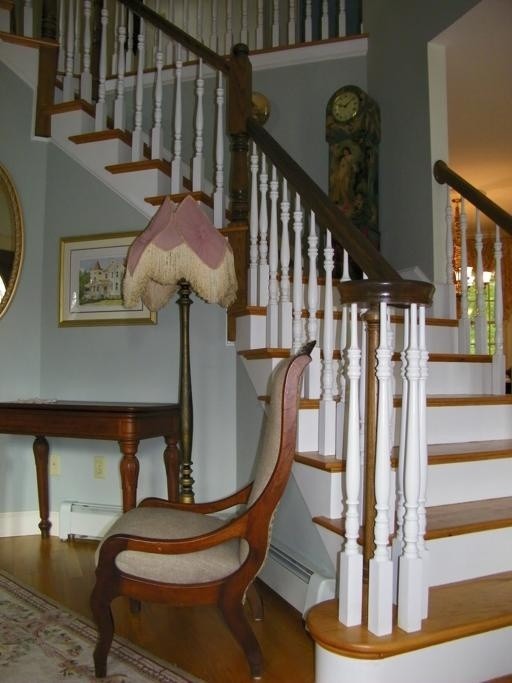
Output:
[452,197,492,298]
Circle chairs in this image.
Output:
[85,339,319,678]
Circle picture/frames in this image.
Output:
[57,229,158,326]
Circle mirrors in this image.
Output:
[0,161,26,318]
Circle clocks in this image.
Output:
[324,84,381,250]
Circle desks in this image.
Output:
[0,399,179,539]
